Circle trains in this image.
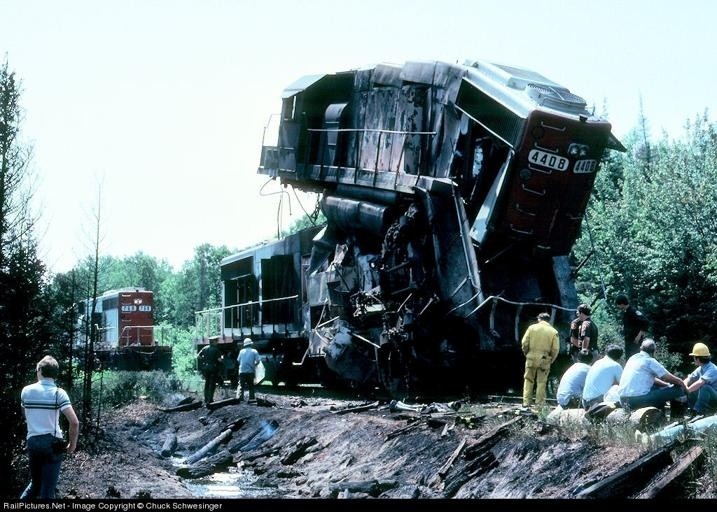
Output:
[62,283,173,371]
[192,56,629,417]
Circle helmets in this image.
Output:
[244,338,253,346]
[688,342,711,357]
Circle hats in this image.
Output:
[640,339,655,351]
[208,335,220,342]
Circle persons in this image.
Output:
[519,312,561,408]
[576,304,600,365]
[614,295,650,362]
[555,348,594,409]
[195,336,224,406]
[233,336,261,404]
[580,344,623,411]
[681,341,716,419]
[616,335,689,424]
[567,303,592,362]
[17,354,80,501]
